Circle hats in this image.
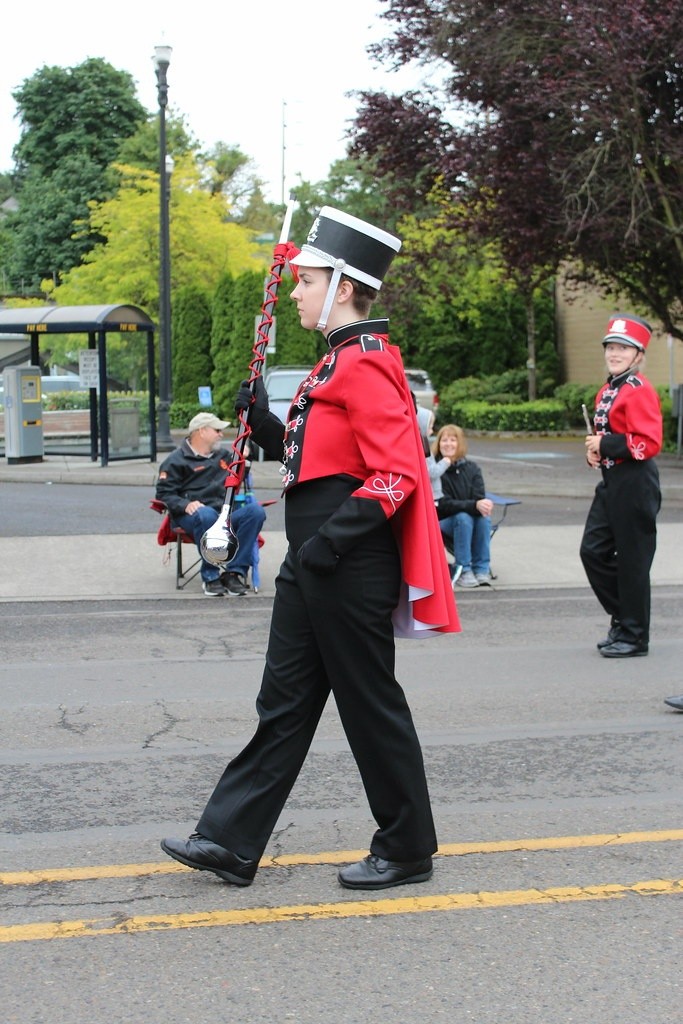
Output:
[289,206,402,291]
[188,412,231,434]
[601,313,654,354]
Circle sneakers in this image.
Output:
[201,578,226,596]
[220,575,247,596]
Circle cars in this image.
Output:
[248,363,319,463]
[40,375,99,399]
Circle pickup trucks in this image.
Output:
[400,369,438,416]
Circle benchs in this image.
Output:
[0,408,91,448]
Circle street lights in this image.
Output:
[149,38,178,453]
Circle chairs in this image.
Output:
[440,491,523,580]
[148,499,277,589]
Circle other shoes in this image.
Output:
[474,573,492,588]
[455,570,479,588]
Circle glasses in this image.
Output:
[214,429,222,433]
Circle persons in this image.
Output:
[430,423,494,588]
[579,313,663,659]
[155,412,267,597]
[159,205,461,891]
[416,406,451,508]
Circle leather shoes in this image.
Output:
[597,632,649,657]
[664,694,683,709]
[337,852,434,890]
[160,831,259,886]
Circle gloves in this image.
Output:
[296,534,340,577]
[235,374,269,426]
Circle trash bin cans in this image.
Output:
[110,399,140,451]
